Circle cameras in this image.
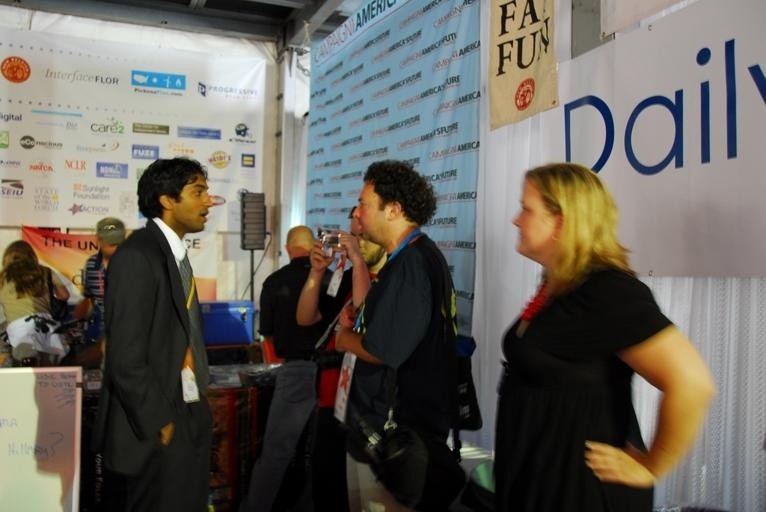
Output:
[322,234,341,247]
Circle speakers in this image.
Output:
[241,192,265,250]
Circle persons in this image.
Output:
[296,206,387,512]
[493,163,714,512]
[247,226,334,512]
[74,218,126,366]
[0,241,70,366]
[103,158,213,512]
[335,159,456,512]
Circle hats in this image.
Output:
[97,217,125,244]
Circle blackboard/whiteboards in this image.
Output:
[0,366,82,512]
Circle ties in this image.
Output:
[179,250,212,398]
